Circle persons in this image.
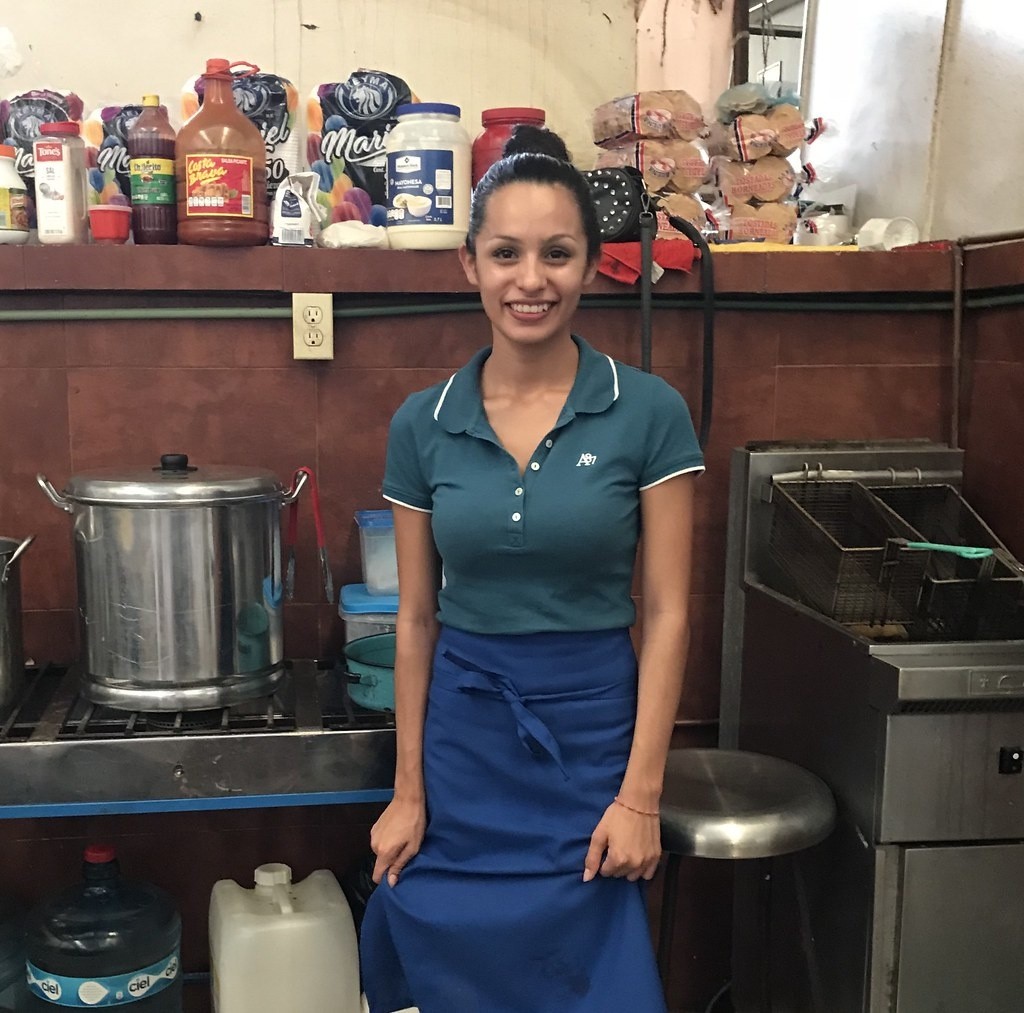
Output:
[359,122,707,1013]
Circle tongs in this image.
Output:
[284,466,334,606]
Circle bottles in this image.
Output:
[385,103,471,248]
[0,145,31,247]
[26,846,182,1013]
[178,58,270,244]
[131,97,178,245]
[471,107,547,196]
[210,863,365,1013]
[31,123,84,244]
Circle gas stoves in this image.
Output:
[0,660,409,806]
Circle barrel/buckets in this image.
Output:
[0,533,33,714]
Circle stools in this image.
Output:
[658,746,838,1012]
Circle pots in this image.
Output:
[338,631,397,713]
[36,456,310,712]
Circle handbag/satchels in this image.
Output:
[580,167,658,244]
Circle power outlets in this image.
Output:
[292,292,333,359]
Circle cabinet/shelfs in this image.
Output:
[891,842,1024,1012]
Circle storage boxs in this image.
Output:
[338,583,398,639]
[354,509,446,595]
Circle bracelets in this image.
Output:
[613,790,661,818]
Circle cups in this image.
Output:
[88,205,131,244]
